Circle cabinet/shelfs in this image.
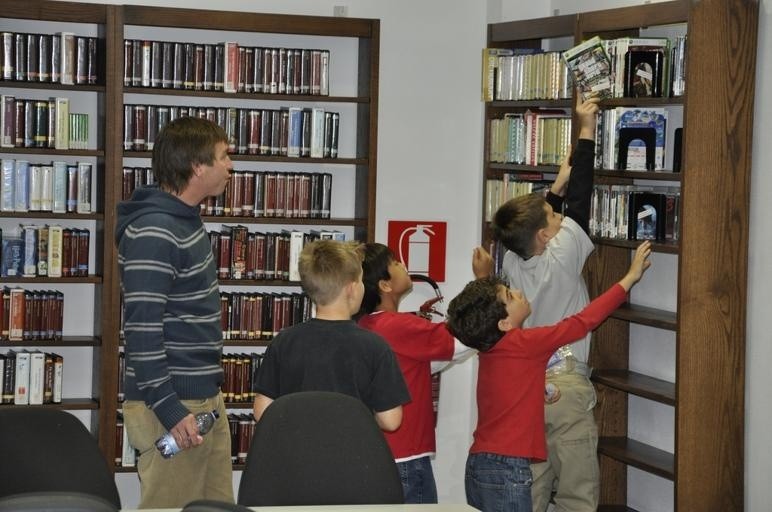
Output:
[579,1,756,512]
[107,3,380,471]
[482,14,579,262]
[0,0,107,460]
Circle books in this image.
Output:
[114,39,338,467]
[0,30,102,406]
[479,36,688,248]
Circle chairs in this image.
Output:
[235,391,409,503]
[0,407,122,510]
[184,499,253,511]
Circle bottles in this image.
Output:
[156,410,221,457]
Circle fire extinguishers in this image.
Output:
[404,274,444,428]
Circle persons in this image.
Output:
[253,238,456,506]
[445,90,651,512]
[117,119,237,510]
